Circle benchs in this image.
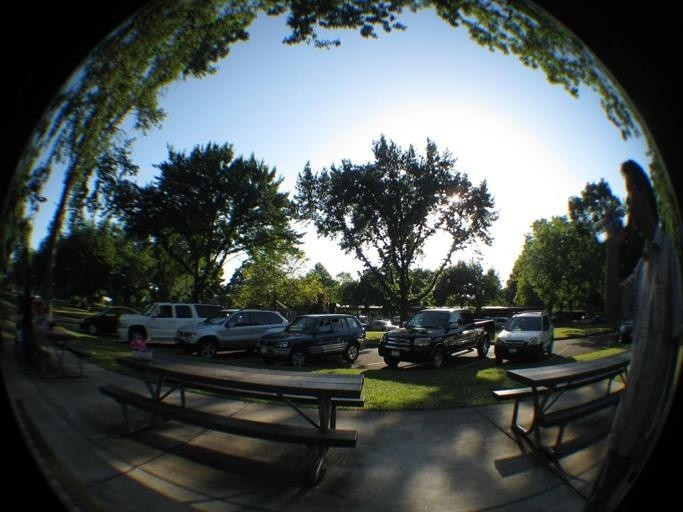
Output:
[491,367,624,434]
[543,388,629,459]
[49,338,97,379]
[25,339,49,377]
[98,383,355,488]
[140,372,364,430]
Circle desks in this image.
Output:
[116,350,364,456]
[508,349,630,449]
[40,320,98,378]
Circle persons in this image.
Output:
[598,160,660,320]
